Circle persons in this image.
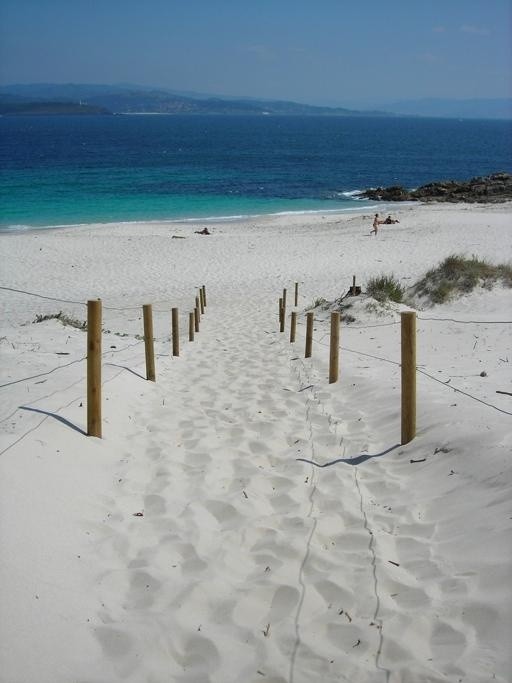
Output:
[370,214,385,236]
